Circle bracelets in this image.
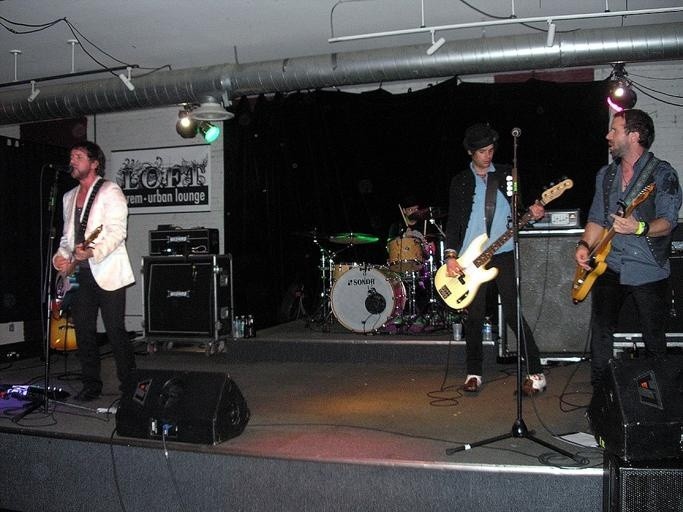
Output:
[576,240,588,249]
[635,221,649,236]
[445,251,456,259]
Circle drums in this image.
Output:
[332,264,357,283]
[331,262,407,334]
[389,236,425,273]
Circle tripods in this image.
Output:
[9,245,83,424]
[310,277,335,329]
[406,274,459,336]
[447,223,583,465]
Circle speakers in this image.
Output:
[601,450,683,511]
[587,351,683,462]
[114,367,252,446]
[142,255,232,339]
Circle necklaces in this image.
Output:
[623,175,633,187]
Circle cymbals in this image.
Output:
[330,233,379,244]
[409,207,446,220]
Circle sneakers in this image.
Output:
[77,380,100,400]
[464,375,482,392]
[521,372,547,396]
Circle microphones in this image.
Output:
[511,125,523,138]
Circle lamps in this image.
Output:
[605,59,637,114]
[23,65,136,104]
[421,19,559,56]
[174,93,221,145]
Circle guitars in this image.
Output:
[55,226,102,303]
[571,184,656,305]
[49,304,84,350]
[434,176,574,308]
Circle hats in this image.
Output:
[464,124,499,151]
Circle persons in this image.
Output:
[52,141,136,401]
[575,109,683,422]
[444,128,547,396]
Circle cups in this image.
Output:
[452,323,462,340]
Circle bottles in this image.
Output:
[6,387,26,396]
[232,314,255,338]
[482,317,492,341]
[630,342,639,360]
